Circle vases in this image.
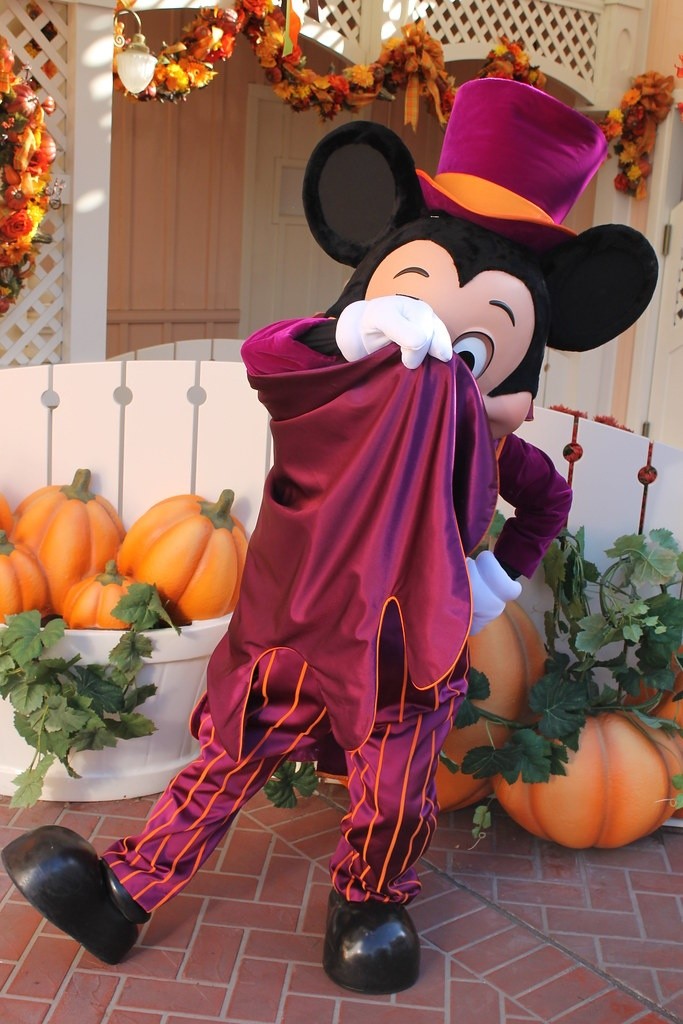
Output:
[0,607,239,804]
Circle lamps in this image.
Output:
[114,8,158,97]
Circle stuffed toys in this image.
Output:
[1,77,660,994]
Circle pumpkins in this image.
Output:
[0,469,247,629]
[311,598,683,850]
